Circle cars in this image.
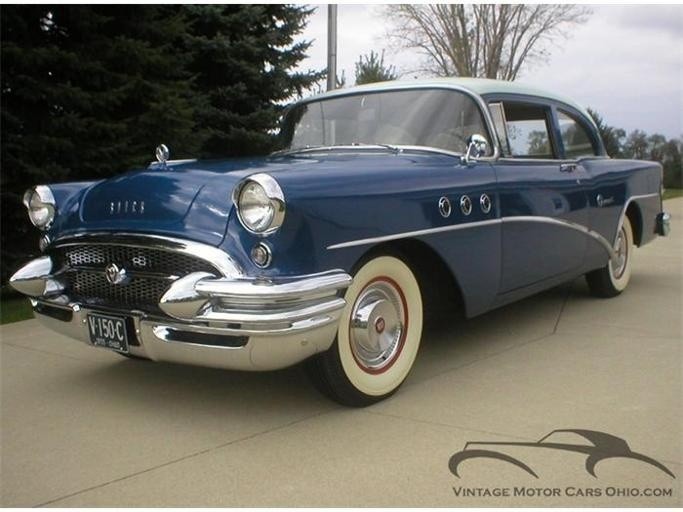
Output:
[5,75,671,407]
